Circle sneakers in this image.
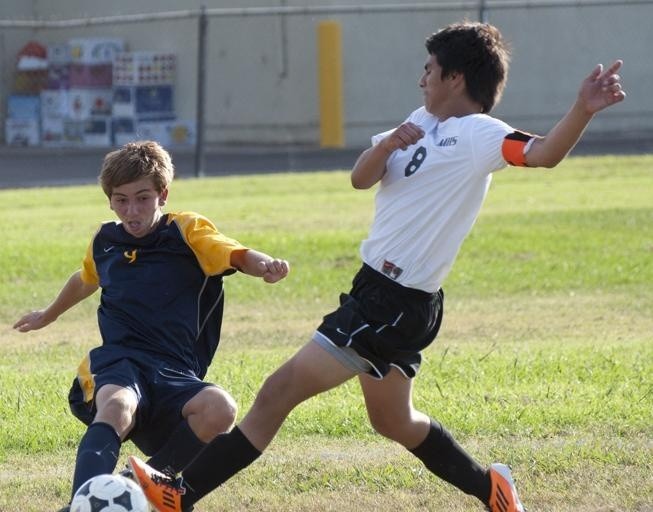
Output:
[127,455,194,512]
[487,463,524,512]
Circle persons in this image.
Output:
[129,21,626,512]
[13,140,288,512]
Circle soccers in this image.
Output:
[69,473,150,512]
